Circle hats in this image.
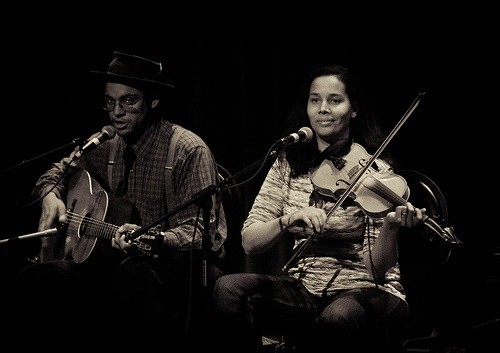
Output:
[88,50,173,90]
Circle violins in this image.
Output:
[311,141,461,246]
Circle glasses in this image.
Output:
[101,98,139,112]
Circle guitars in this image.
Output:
[39,168,164,264]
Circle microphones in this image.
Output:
[72,125,116,160]
[275,127,313,147]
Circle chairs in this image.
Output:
[217,162,448,353]
